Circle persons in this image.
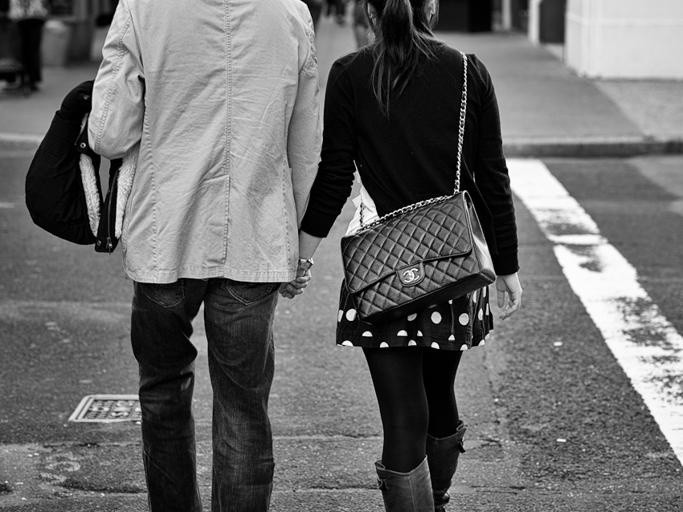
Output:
[302,1,374,49]
[278,7,524,512]
[7,1,48,90]
[87,1,322,512]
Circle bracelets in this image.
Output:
[291,254,316,267]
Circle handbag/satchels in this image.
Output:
[340,188,496,321]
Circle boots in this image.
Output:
[374,457,435,511]
[428,419,466,512]
